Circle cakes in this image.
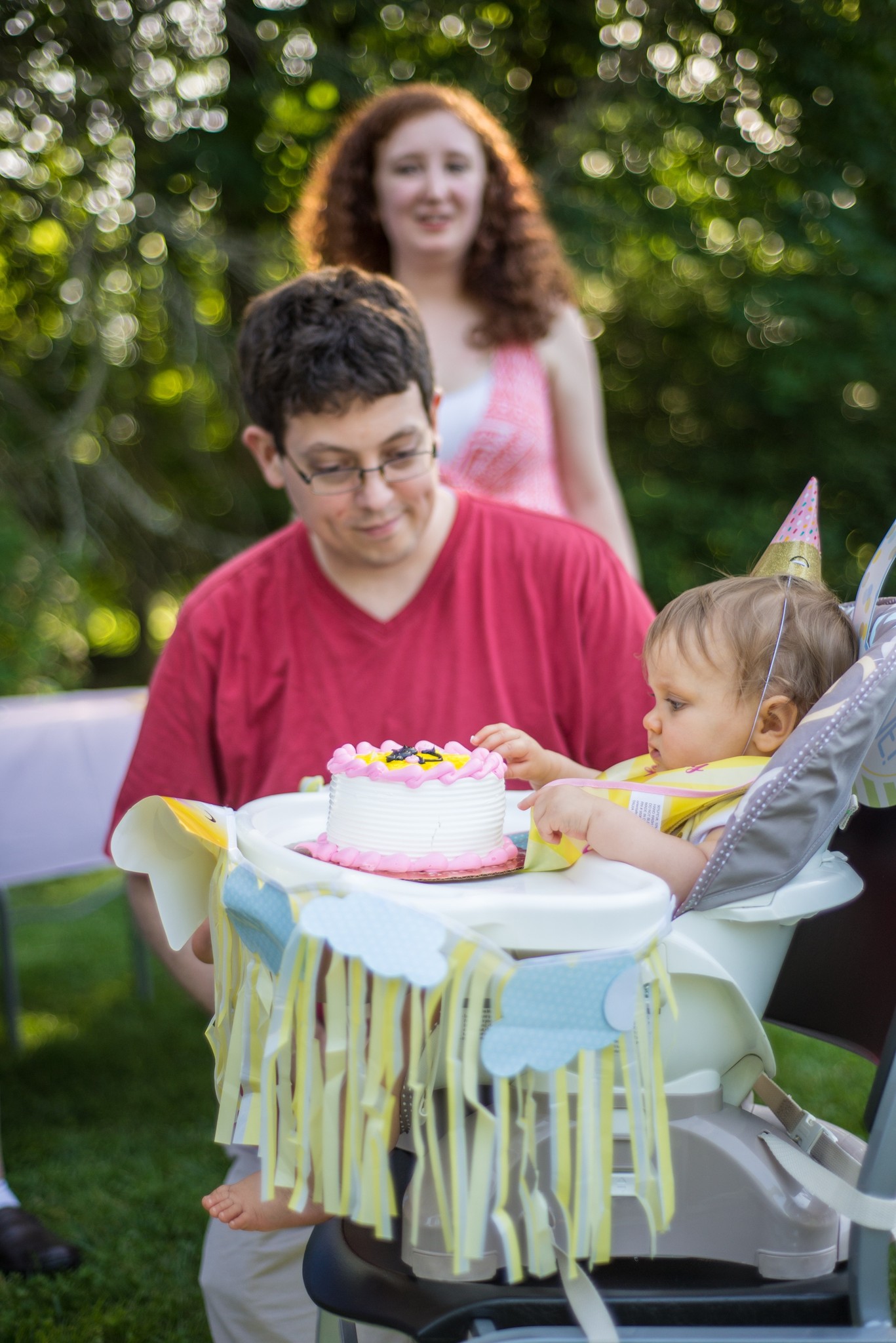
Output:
[301,740,517,873]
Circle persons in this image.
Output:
[289,84,647,602]
[203,573,861,1233]
[101,266,656,1342]
[0,1173,82,1272]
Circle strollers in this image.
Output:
[231,513,896,1342]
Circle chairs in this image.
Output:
[234,598,896,1342]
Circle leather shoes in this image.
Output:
[1,1206,75,1269]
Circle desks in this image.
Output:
[0,686,152,1006]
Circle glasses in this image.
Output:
[282,420,437,494]
